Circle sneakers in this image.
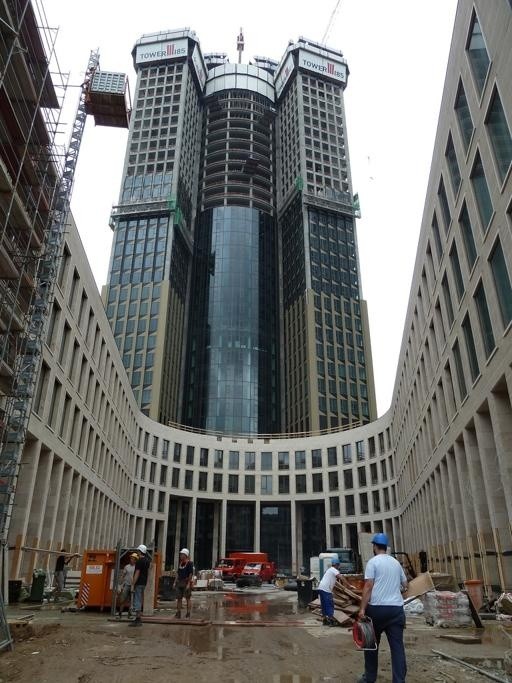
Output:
[129,618,142,627]
[185,613,191,620]
[116,613,122,619]
[321,615,330,624]
[328,616,338,626]
[174,611,181,618]
[127,610,134,619]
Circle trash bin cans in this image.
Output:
[295,577,317,608]
[31,573,46,602]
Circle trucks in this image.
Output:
[214,550,266,583]
[327,549,363,574]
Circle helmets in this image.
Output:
[179,548,190,557]
[332,558,340,565]
[137,544,147,554]
[129,552,139,560]
[370,532,388,545]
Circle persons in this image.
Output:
[118,553,139,619]
[43,549,80,603]
[128,544,151,627]
[173,548,194,618]
[318,558,358,627]
[356,533,409,683]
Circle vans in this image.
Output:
[240,560,281,588]
